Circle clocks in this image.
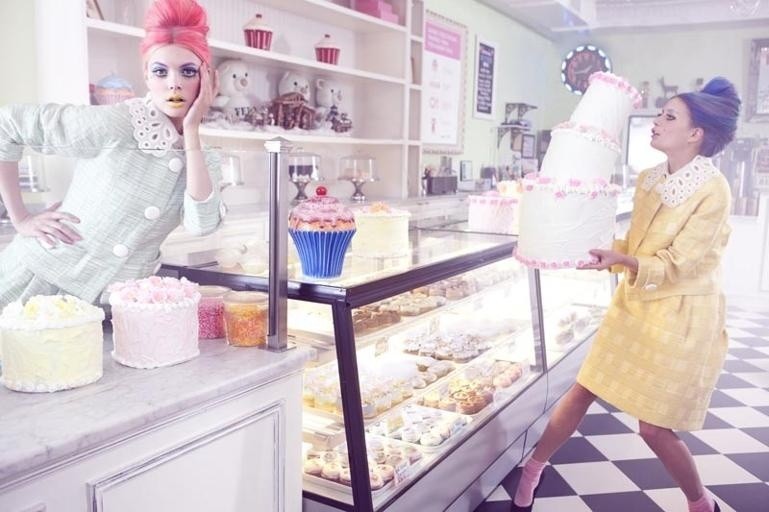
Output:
[560,45,611,96]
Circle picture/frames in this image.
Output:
[745,37,769,125]
[520,134,535,159]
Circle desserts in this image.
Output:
[301,276,541,487]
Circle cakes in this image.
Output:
[0,294,105,393]
[467,179,519,234]
[352,202,412,257]
[109,275,201,369]
[513,71,643,268]
[289,186,357,280]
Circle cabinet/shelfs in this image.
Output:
[34,0,426,243]
[2,367,307,512]
[156,195,636,511]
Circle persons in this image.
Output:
[0,1,224,314]
[510,76,739,512]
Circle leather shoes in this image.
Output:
[511,471,545,511]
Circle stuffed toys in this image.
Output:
[265,69,324,136]
[314,77,355,137]
[211,58,263,131]
